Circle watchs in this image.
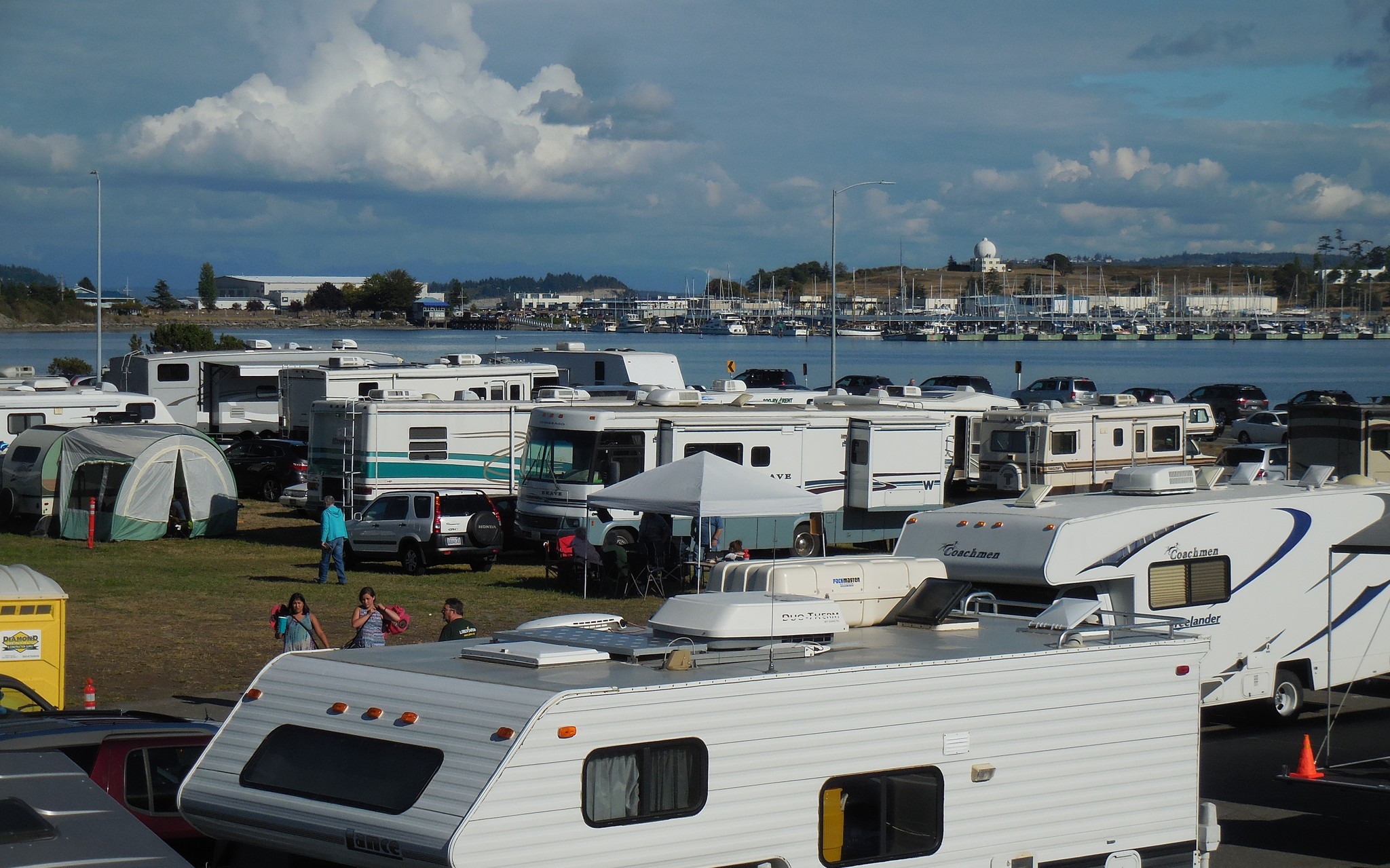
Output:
[713,537,717,541]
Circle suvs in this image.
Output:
[69,374,103,387]
[1177,383,1269,423]
[338,489,505,575]
[1274,390,1360,425]
[732,369,796,388]
[222,438,308,503]
[0,711,231,851]
[1210,442,1288,485]
[917,374,994,396]
[1010,376,1100,406]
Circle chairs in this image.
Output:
[557,534,576,558]
[543,540,563,579]
[622,541,667,604]
[572,547,593,587]
[654,535,692,589]
[598,548,639,598]
[700,550,725,589]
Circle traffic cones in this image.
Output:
[1289,734,1325,780]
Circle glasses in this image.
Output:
[443,607,457,613]
[729,547,732,550]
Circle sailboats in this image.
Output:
[592,262,1390,342]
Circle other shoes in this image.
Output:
[336,582,346,585]
[685,576,697,590]
[313,578,327,584]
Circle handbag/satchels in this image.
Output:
[376,605,410,640]
[342,639,358,649]
[270,604,288,629]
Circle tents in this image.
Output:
[58,423,238,542]
[584,451,826,600]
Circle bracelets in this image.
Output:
[384,607,387,611]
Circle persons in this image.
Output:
[908,378,915,385]
[715,540,745,563]
[95,493,106,512]
[690,517,723,584]
[275,592,330,652]
[638,513,671,544]
[571,527,603,566]
[438,598,477,643]
[351,587,400,649]
[603,532,628,576]
[313,497,348,586]
[181,491,187,497]
[171,492,190,539]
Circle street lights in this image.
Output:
[89,169,102,382]
[831,179,896,388]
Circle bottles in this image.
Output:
[688,553,693,562]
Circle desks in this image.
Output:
[683,561,715,568]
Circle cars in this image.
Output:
[1187,417,1225,443]
[768,384,813,404]
[1231,410,1289,444]
[279,483,308,511]
[1118,388,1176,403]
[812,375,896,396]
